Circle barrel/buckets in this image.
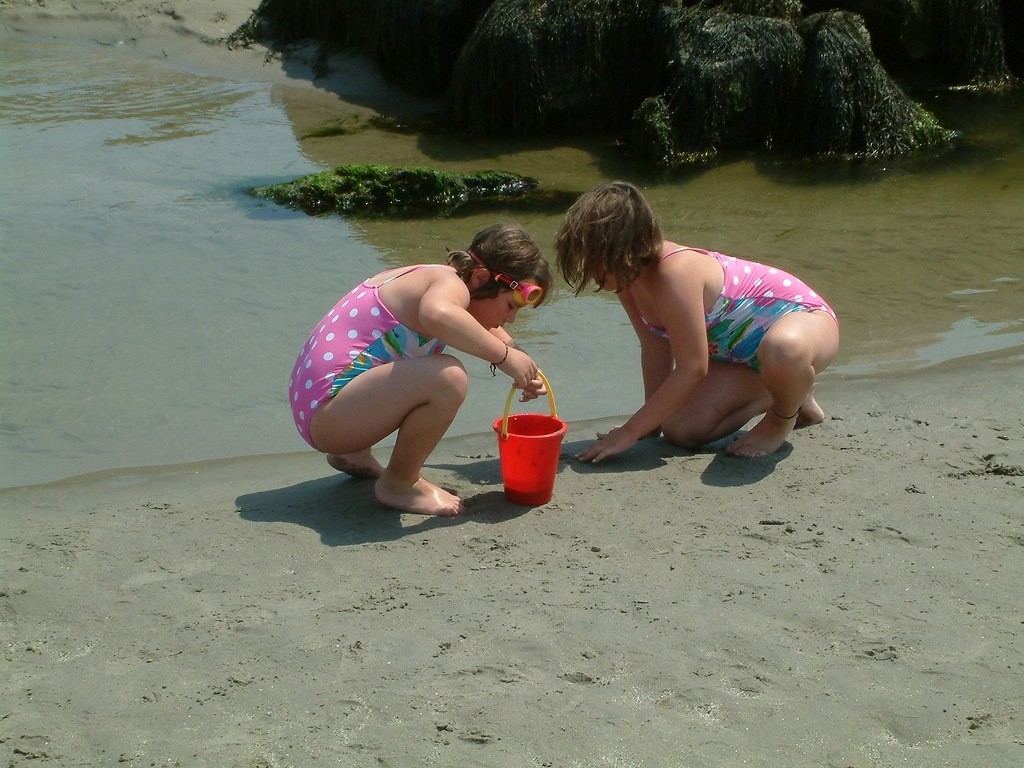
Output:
[492,368,565,506]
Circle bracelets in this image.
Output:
[489,341,508,377]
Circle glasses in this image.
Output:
[514,277,544,305]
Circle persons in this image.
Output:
[554,184,839,460]
[288,225,551,516]
